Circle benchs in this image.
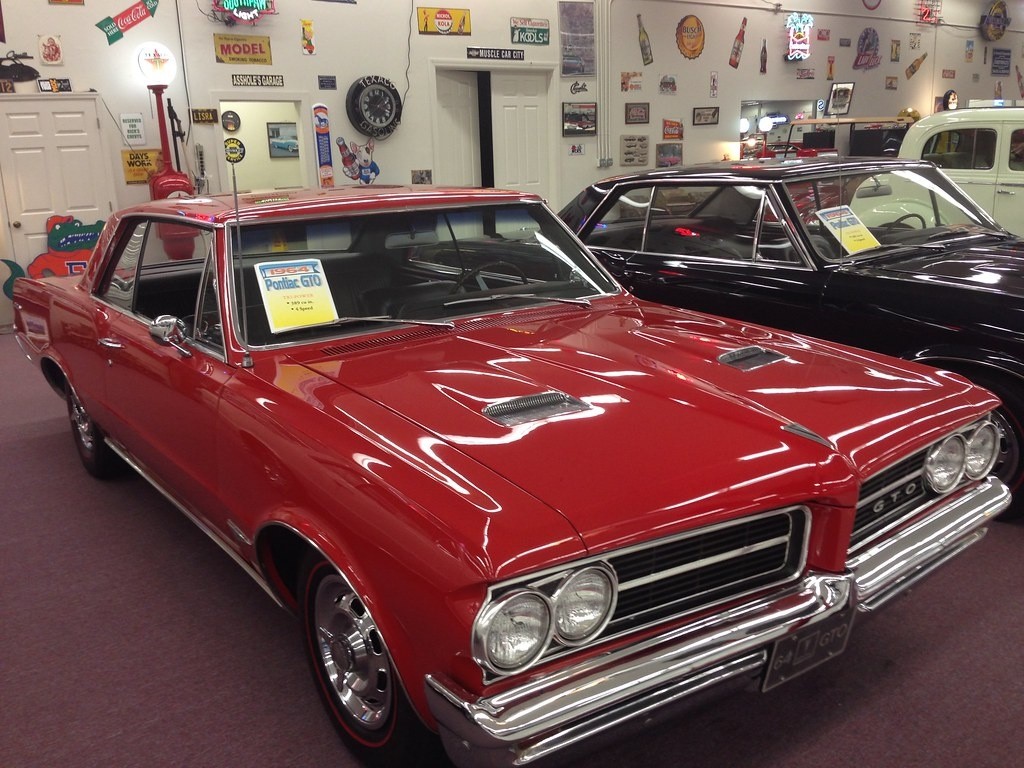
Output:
[590,215,737,259]
[134,252,400,330]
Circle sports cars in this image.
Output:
[434,155,1024,534]
[12,183,1017,768]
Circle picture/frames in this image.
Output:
[562,102,597,137]
[693,107,719,125]
[625,102,649,124]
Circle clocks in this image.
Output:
[346,75,402,137]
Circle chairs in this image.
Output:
[183,304,265,346]
[369,281,464,326]
[767,234,839,263]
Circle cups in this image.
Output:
[891,40,900,62]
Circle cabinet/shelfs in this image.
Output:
[0,92,118,334]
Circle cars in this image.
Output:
[744,143,877,224]
[847,106,1024,237]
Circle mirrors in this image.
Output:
[740,99,817,160]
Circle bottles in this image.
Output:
[906,52,927,80]
[760,39,767,73]
[336,136,361,180]
[1015,65,1024,98]
[827,56,835,80]
[729,18,747,69]
[637,14,654,66]
[995,80,1001,98]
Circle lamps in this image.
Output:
[740,118,749,132]
[759,117,773,131]
